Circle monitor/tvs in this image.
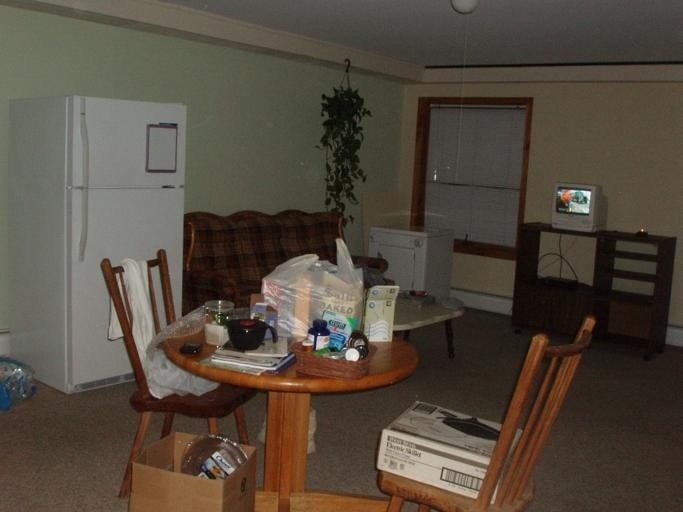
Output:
[552,182,607,232]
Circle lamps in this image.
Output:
[450,0,480,14]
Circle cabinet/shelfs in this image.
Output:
[514,222,676,361]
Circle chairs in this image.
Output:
[378,318,597,512]
[101,248,260,499]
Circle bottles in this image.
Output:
[204,301,236,346]
[302,319,369,362]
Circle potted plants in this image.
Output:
[316,87,372,229]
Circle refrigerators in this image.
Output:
[5,98,186,393]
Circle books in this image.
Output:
[198,335,297,377]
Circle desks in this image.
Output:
[393,295,465,360]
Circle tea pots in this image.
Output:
[220,319,276,350]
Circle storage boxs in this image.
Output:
[130,434,257,512]
[375,400,523,502]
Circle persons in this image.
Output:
[556,189,573,214]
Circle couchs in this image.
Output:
[181,210,389,314]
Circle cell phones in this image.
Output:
[179,341,202,354]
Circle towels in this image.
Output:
[107,257,156,373]
